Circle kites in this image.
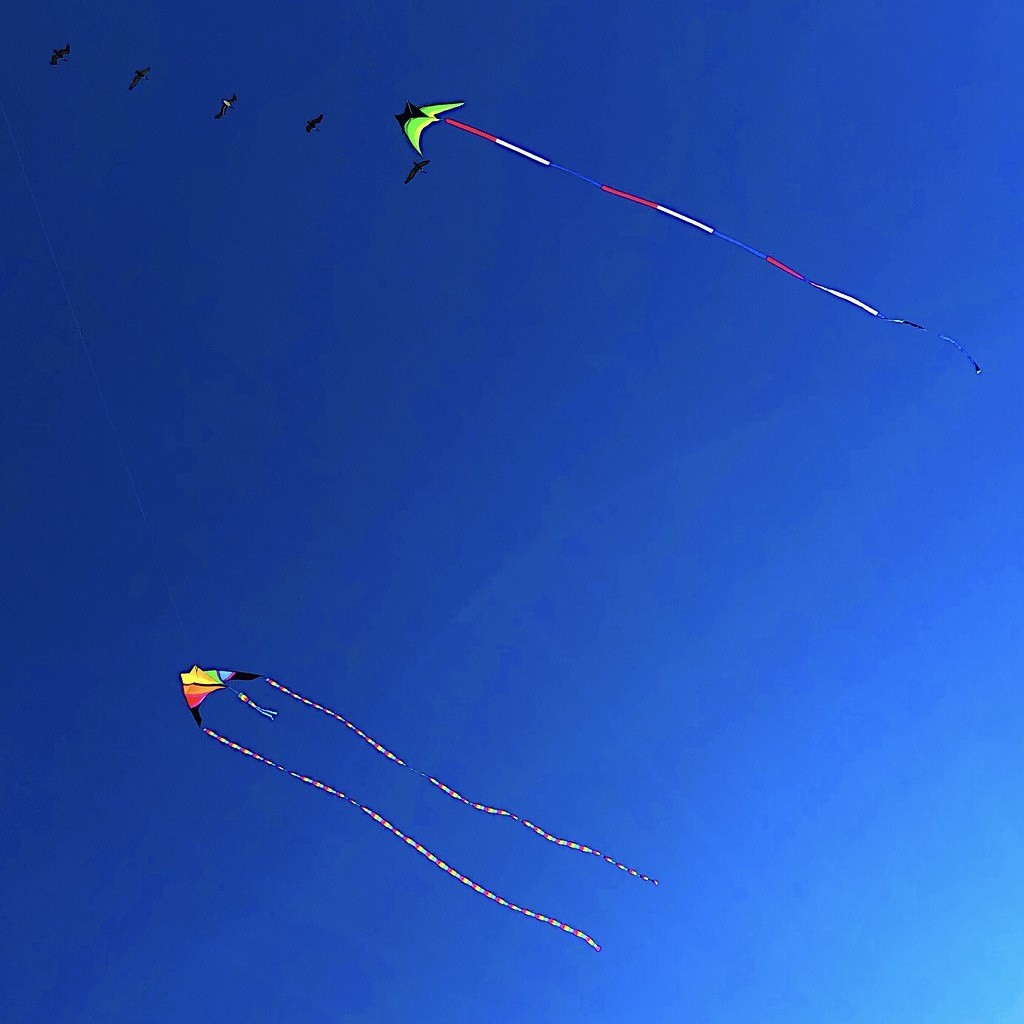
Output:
[180,664,659,952]
[394,100,982,375]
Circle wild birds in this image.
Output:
[405,159,430,184]
[305,113,324,135]
[128,66,152,90]
[48,45,71,66]
[214,94,238,119]
